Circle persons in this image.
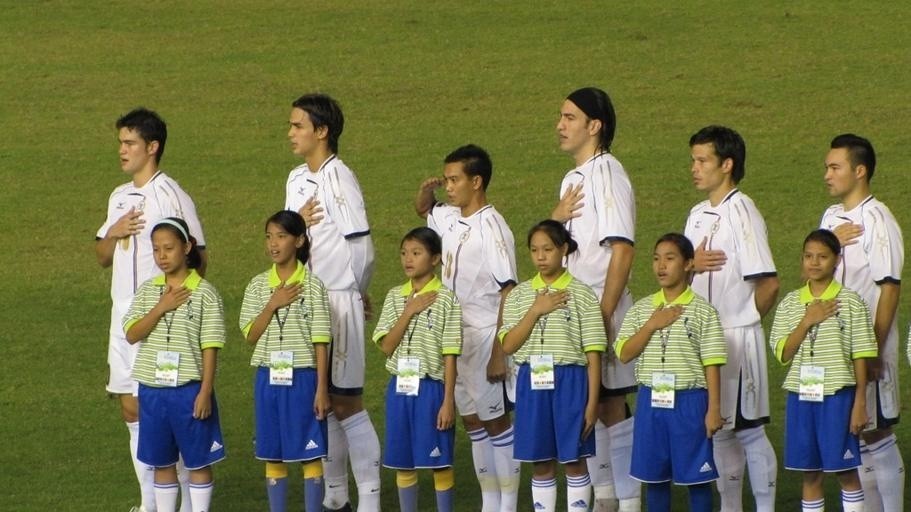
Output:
[93,107,207,512]
[417,145,521,512]
[495,221,609,512]
[122,216,226,512]
[238,210,332,512]
[611,232,729,511]
[769,229,880,512]
[550,87,642,511]
[284,93,382,512]
[375,225,464,512]
[681,124,780,512]
[818,133,908,511]
